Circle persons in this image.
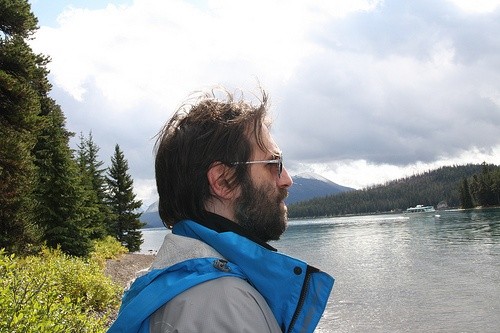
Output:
[106,78,336,332]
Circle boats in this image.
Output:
[404,204,435,214]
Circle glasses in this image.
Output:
[220,152,284,178]
